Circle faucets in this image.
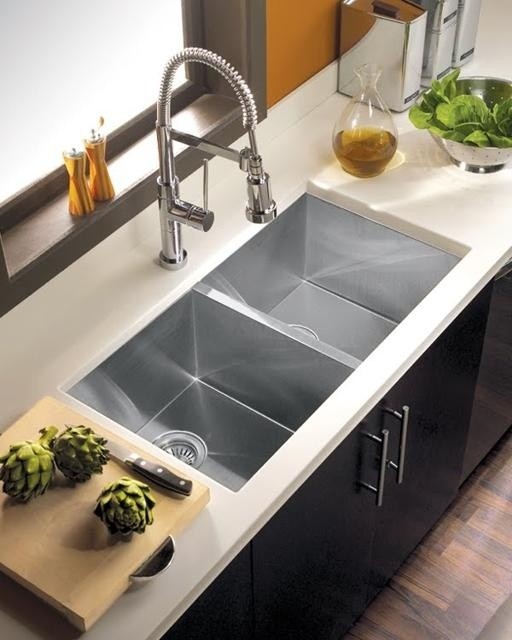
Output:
[155,48,277,223]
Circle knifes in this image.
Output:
[64,422,192,496]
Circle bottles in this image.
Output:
[332,59,399,179]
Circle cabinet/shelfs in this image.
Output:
[250,277,495,639]
[461,258,511,489]
[160,542,250,638]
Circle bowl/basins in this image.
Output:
[422,76,512,173]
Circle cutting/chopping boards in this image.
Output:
[1,397,210,633]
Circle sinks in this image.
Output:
[200,192,471,361]
[57,288,354,492]
